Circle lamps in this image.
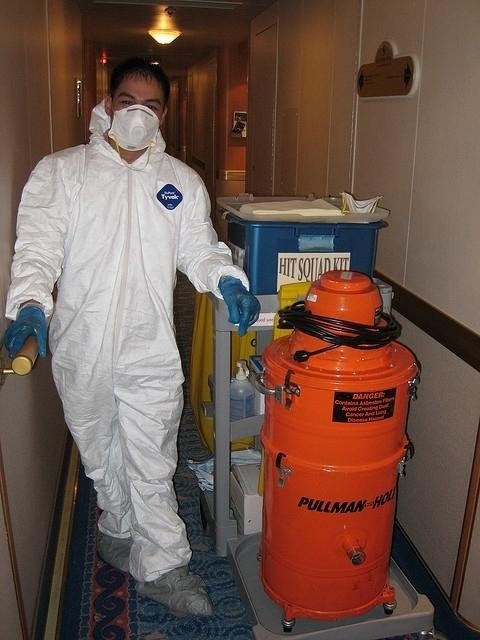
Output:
[146,26,184,47]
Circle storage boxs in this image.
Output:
[214,192,388,297]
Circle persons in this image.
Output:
[4,59,261,618]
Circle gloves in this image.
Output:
[5,304,47,359]
[217,275,260,337]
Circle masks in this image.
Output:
[109,104,160,151]
